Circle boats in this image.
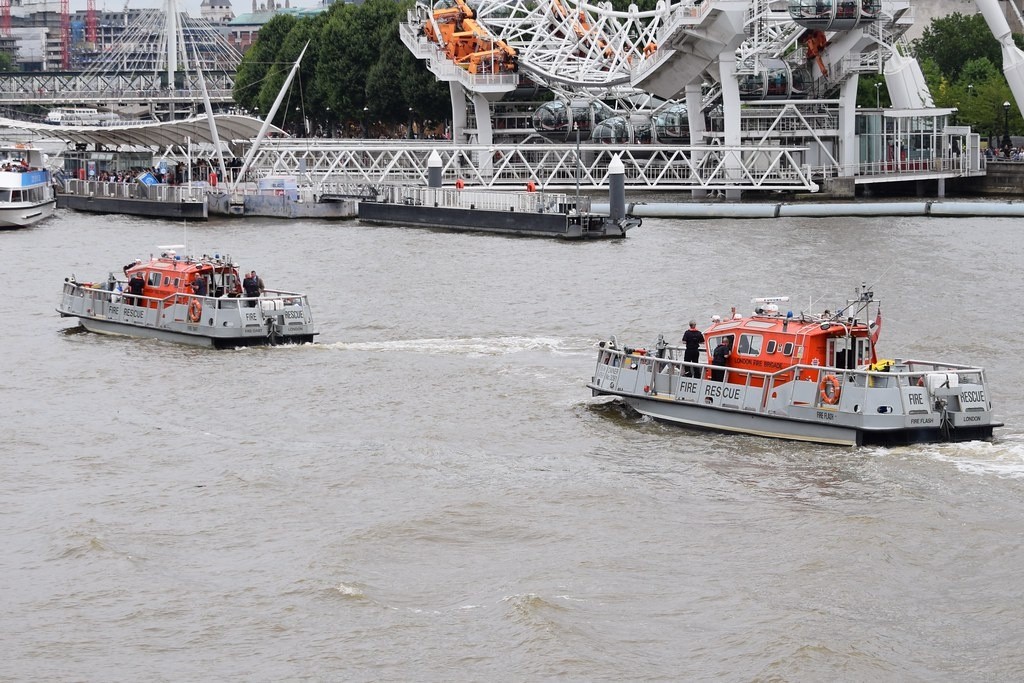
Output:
[585,281,1005,447]
[0,144,58,228]
[55,244,320,348]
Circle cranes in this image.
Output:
[60,0,70,72]
[0,0,59,37]
[87,0,97,50]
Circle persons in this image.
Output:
[130,272,265,308]
[682,320,705,379]
[711,338,731,382]
[980,145,1024,162]
[0,158,251,186]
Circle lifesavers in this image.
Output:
[189,300,201,321]
[820,375,839,405]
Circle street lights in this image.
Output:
[296,107,302,138]
[1001,100,1013,157]
[229,107,259,119]
[528,104,534,128]
[701,83,709,95]
[363,107,369,139]
[968,85,973,108]
[326,107,332,138]
[874,83,882,108]
[407,107,415,139]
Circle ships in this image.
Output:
[42,107,98,125]
